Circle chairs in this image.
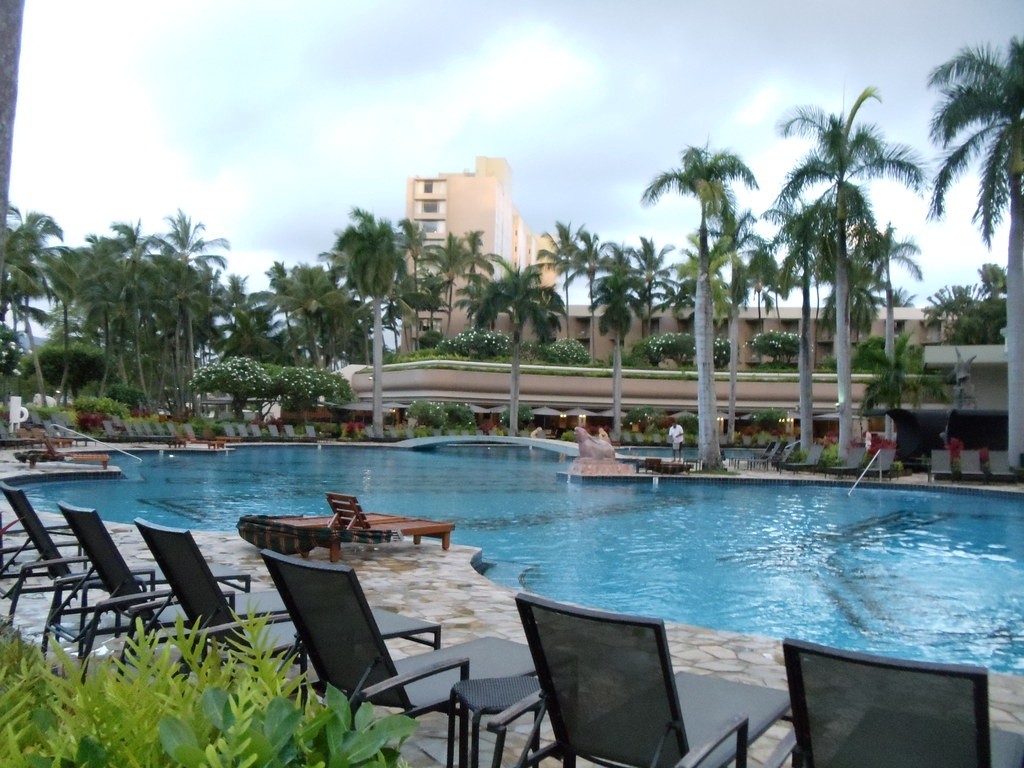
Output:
[781,637,1024,768]
[0,481,252,647]
[117,517,443,698]
[1,410,1023,485]
[0,510,90,570]
[261,548,540,766]
[487,592,798,767]
[34,499,289,686]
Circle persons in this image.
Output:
[668,420,685,462]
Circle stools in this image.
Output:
[447,676,569,768]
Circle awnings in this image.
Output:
[669,410,865,436]
[339,399,630,430]
